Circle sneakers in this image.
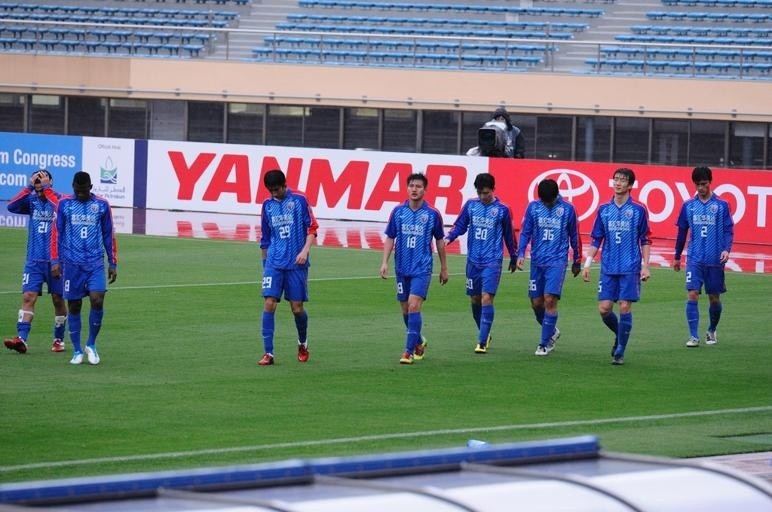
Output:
[297,336,309,362]
[548,327,562,352]
[611,348,626,366]
[483,333,494,349]
[51,337,65,352]
[684,334,702,347]
[84,344,101,366]
[609,336,621,358]
[3,336,28,353]
[414,337,427,360]
[398,350,415,366]
[474,342,489,356]
[259,351,275,365]
[533,343,549,357]
[70,350,86,367]
[705,327,719,345]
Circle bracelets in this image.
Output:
[584,256,593,267]
[261,259,267,267]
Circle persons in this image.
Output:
[258,170,319,365]
[444,174,517,353]
[583,168,653,365]
[516,180,582,356]
[4,170,74,352]
[483,106,525,158]
[51,171,119,365]
[378,172,448,364]
[673,166,733,347]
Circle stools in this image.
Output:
[0,0,249,59]
[586,0,772,76]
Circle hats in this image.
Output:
[493,107,512,130]
